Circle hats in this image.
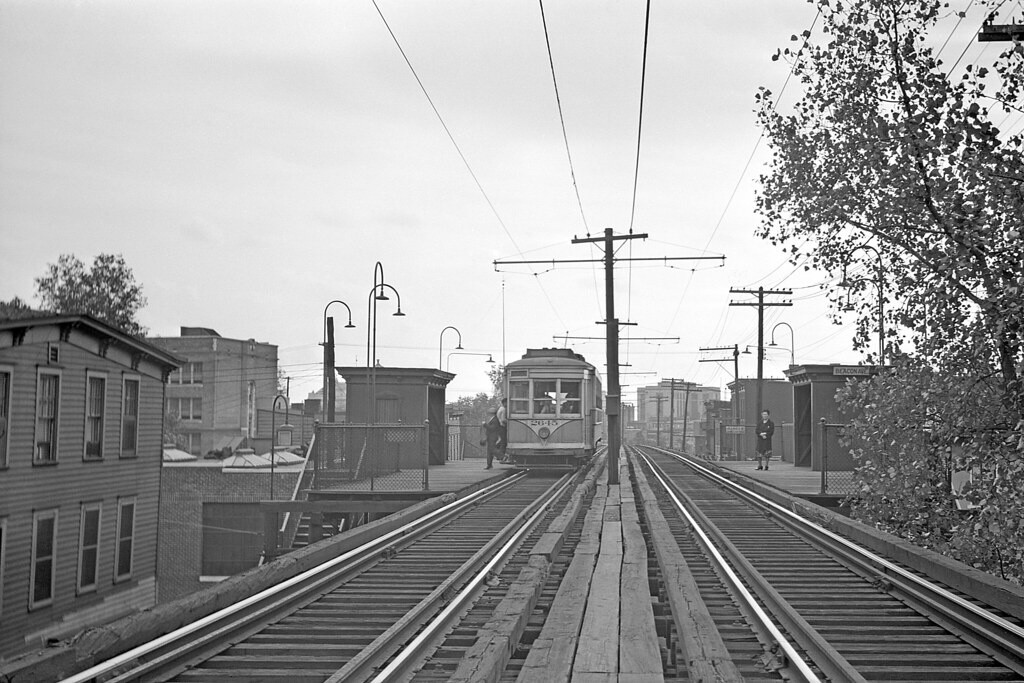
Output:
[487,408,498,413]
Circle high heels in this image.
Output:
[765,466,768,470]
[755,466,763,470]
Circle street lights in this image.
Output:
[842,278,883,366]
[373,260,390,474]
[439,325,465,370]
[270,392,295,561]
[322,300,358,469]
[366,283,408,490]
[447,352,497,373]
[769,321,795,364]
[836,245,884,364]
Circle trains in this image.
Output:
[503,347,603,473]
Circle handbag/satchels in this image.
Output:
[480,438,487,445]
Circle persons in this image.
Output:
[755,410,774,470]
[482,398,507,470]
[534,391,578,414]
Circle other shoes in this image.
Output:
[502,456,507,462]
[484,465,492,469]
